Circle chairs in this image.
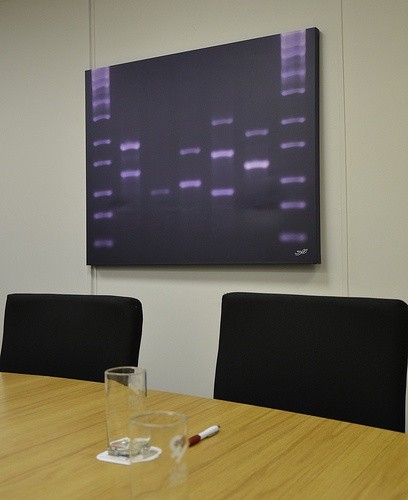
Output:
[0,293,143,383]
[212,292,408,433]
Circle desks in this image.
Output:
[0,370,408,500]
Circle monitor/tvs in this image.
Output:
[85,27,321,266]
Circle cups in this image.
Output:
[129,411,188,500]
[104,367,151,457]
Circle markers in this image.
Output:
[189,424,221,446]
